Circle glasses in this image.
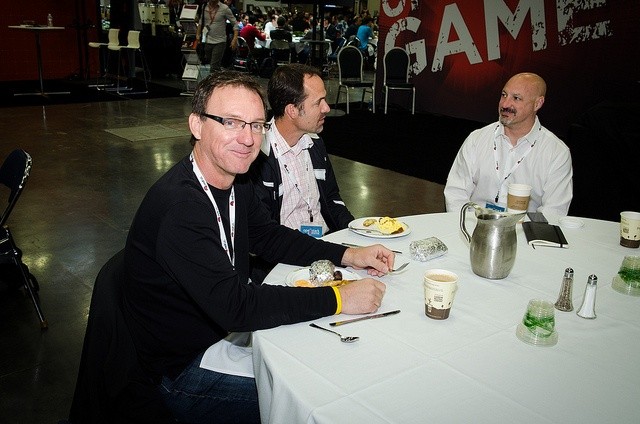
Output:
[201,111,271,134]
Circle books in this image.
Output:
[521,220,569,249]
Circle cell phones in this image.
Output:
[528,211,548,224]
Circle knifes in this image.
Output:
[331,310,399,326]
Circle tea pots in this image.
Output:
[460,202,526,279]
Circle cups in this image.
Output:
[423,269,458,320]
[515,300,559,346]
[506,184,532,222]
[619,211,640,249]
[610,255,636,295]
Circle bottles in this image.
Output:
[554,267,574,313]
[575,275,598,321]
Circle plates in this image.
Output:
[285,266,362,287]
[348,216,412,239]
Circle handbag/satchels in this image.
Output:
[195,3,211,64]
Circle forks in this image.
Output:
[388,262,410,274]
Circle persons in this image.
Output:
[223,0,237,58]
[224,0,238,19]
[444,71,574,217]
[338,9,380,63]
[196,0,239,74]
[122,70,395,412]
[239,8,337,57]
[262,65,355,237]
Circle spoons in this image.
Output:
[309,322,359,343]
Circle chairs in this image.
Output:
[86,28,129,90]
[0,146,50,332]
[328,36,347,62]
[381,47,417,116]
[269,39,293,68]
[235,36,256,59]
[104,30,150,96]
[334,44,377,115]
